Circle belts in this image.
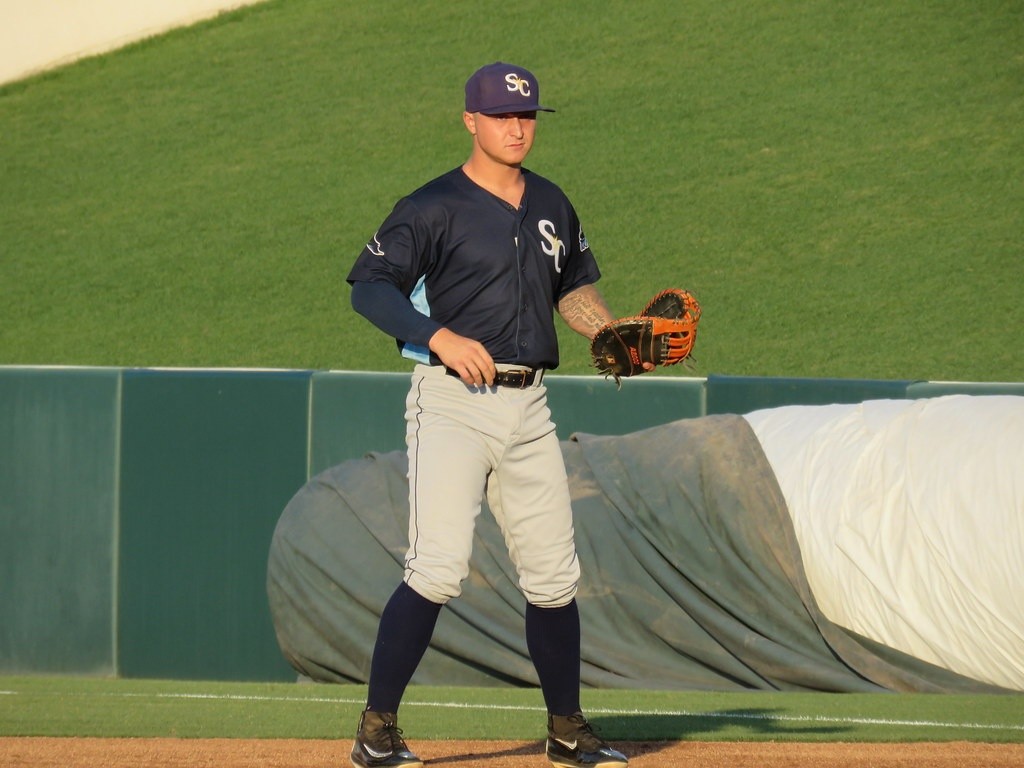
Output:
[446,366,545,388]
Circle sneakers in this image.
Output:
[546,705,628,768]
[350,710,425,768]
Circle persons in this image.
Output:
[346,62,656,768]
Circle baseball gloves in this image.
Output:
[589,287,703,392]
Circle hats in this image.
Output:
[465,61,556,116]
